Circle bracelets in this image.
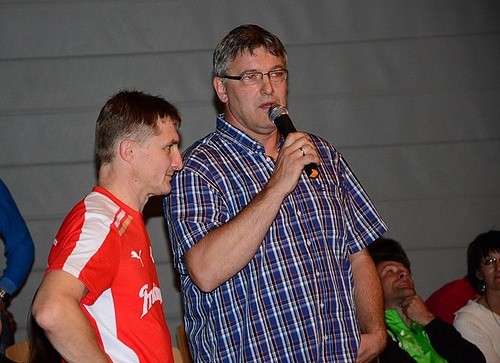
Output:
[0,288,13,304]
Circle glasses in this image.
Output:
[221,69,288,85]
[484,257,497,265]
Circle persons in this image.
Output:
[453,230,500,363]
[367,237,486,363]
[30,90,184,363]
[162,24,389,363]
[0,179,36,363]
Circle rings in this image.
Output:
[300,147,305,156]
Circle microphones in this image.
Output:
[268,104,320,179]
